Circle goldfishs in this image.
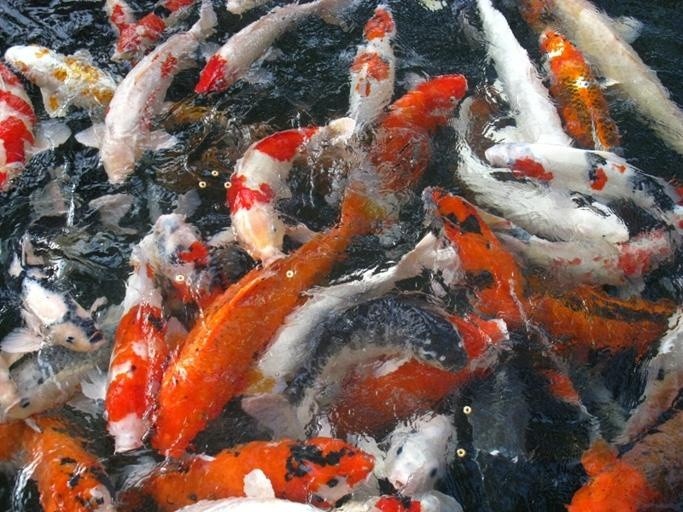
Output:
[0,0,683,512]
